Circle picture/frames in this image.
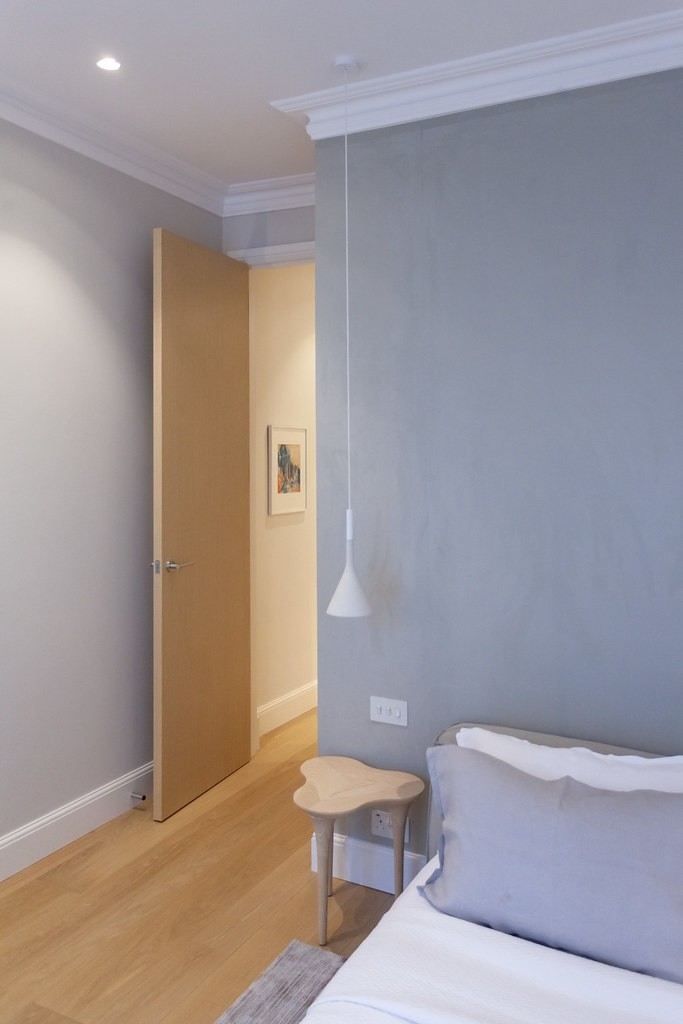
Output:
[268,425,308,517]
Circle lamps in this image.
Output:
[328,59,371,620]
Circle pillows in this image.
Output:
[420,726,682,982]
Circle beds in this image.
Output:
[296,723,682,1024]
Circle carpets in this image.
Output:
[213,937,346,1024]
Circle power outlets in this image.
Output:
[370,810,409,845]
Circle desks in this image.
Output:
[294,754,426,946]
